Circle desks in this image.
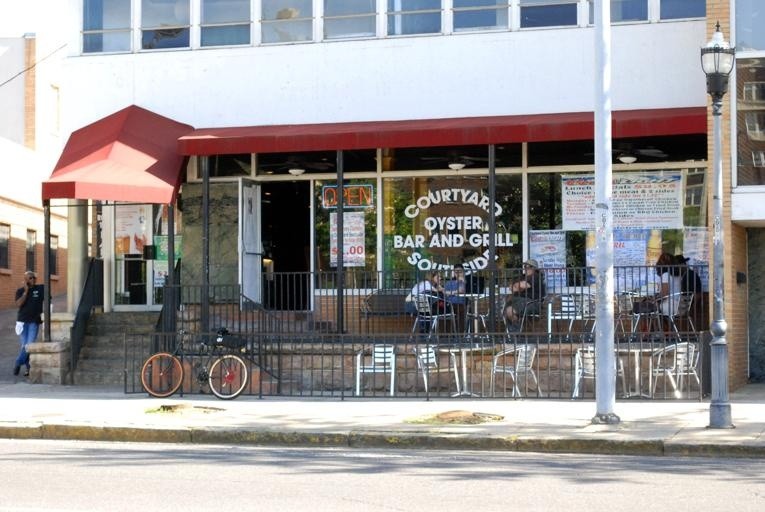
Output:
[614,343,669,399]
[435,344,496,397]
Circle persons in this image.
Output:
[639,253,701,340]
[503,259,546,334]
[13,270,44,376]
[444,264,472,338]
[404,271,465,341]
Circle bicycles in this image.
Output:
[141,327,249,400]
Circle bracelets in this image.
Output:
[23,293,27,296]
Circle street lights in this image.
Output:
[699,19,738,431]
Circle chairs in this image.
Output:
[492,343,544,399]
[545,289,699,340]
[406,291,546,339]
[356,344,397,398]
[641,340,702,399]
[413,344,460,398]
[572,348,628,399]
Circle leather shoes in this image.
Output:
[14,365,30,377]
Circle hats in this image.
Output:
[522,260,538,267]
[673,255,690,264]
